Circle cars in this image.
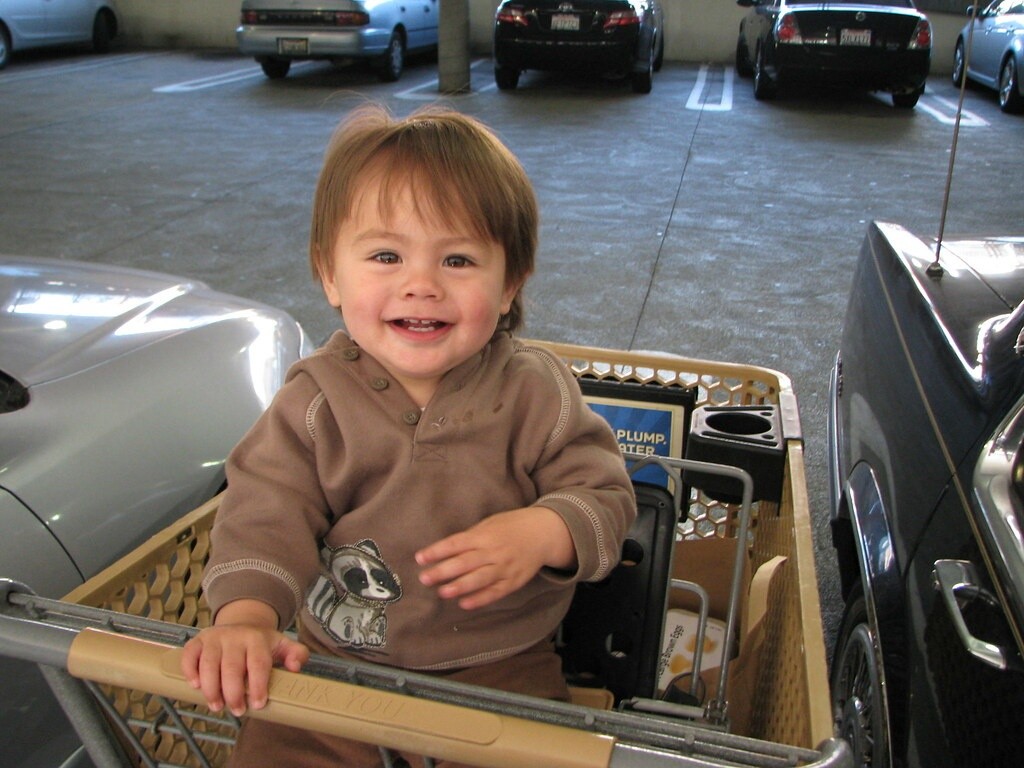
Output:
[823,218,1024,767]
[0,0,122,70]
[234,0,440,84]
[0,252,311,766]
[952,0,1024,113]
[735,0,933,112]
[493,0,664,94]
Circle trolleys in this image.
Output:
[0,337,855,767]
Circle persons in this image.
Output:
[179,111,638,768]
